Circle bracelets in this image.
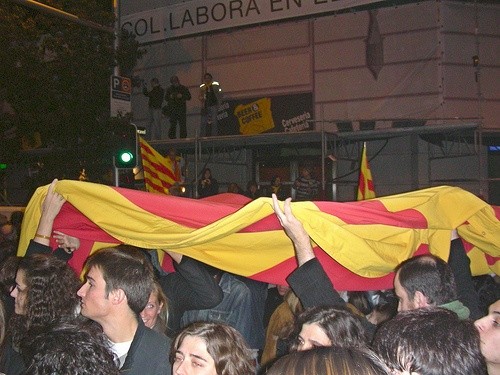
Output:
[34,234,51,243]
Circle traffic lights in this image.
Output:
[112,122,138,169]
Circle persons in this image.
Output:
[0,179,500,375]
[198,168,219,199]
[165,76,192,139]
[243,180,261,200]
[142,77,164,140]
[199,73,224,136]
[266,175,290,201]
[294,168,320,201]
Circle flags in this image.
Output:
[357,147,376,201]
[16,180,500,289]
[139,137,181,195]
